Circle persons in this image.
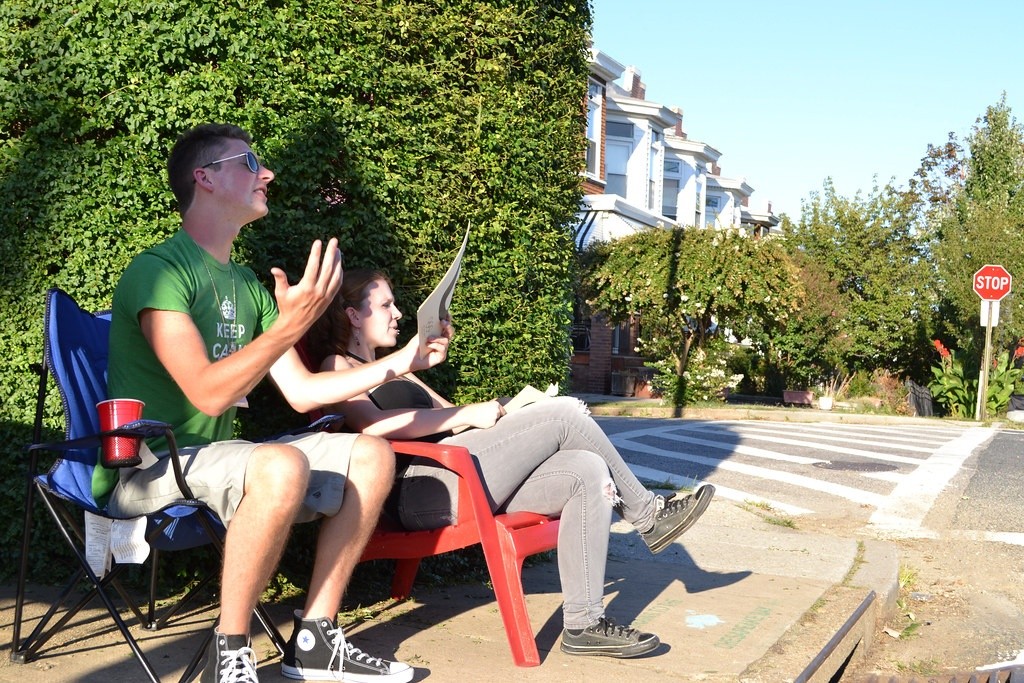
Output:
[92,123,456,683]
[320,269,716,657]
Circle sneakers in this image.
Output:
[280,610,414,683]
[559,617,660,657]
[199,625,257,683]
[640,484,716,555]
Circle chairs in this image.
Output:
[10,287,288,683]
[356,440,560,668]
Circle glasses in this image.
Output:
[193,152,260,184]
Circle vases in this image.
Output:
[818,396,832,410]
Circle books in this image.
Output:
[417,218,471,361]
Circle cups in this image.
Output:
[96,398,145,462]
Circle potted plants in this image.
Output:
[781,390,812,406]
[633,380,659,399]
[611,372,640,398]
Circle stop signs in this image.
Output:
[973,264,1012,301]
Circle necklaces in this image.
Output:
[192,240,236,355]
